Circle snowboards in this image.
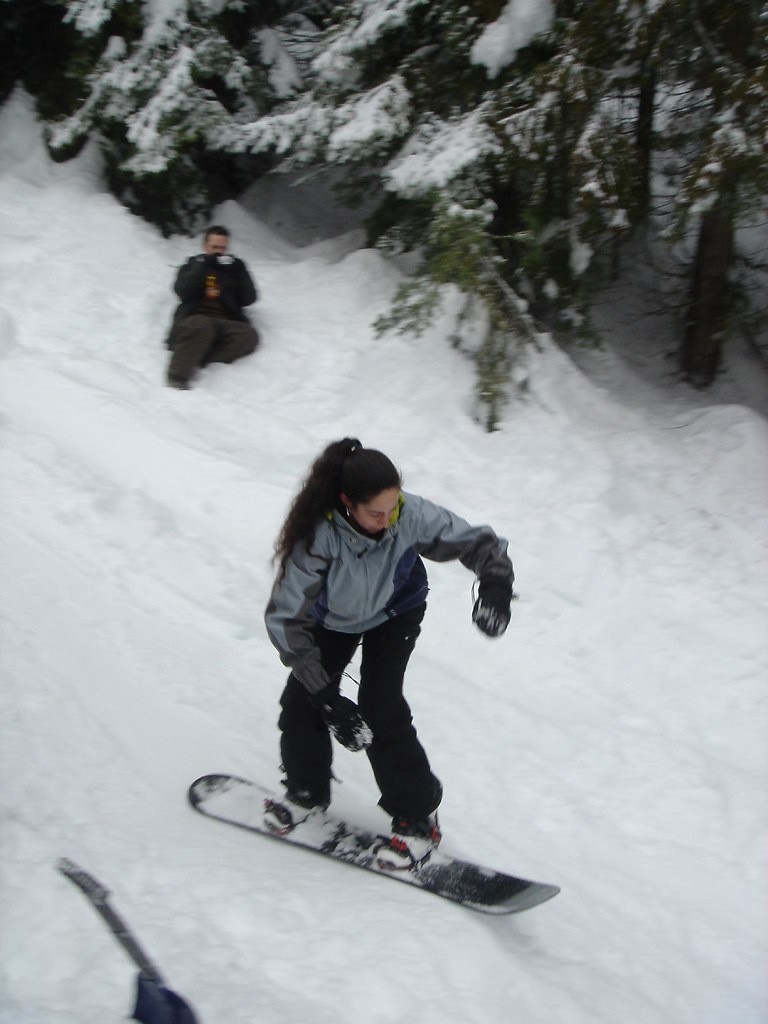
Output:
[187,773,562,916]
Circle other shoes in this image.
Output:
[168,375,190,390]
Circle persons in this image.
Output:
[163,225,260,389]
[263,439,514,869]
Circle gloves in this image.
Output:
[223,255,243,272]
[310,683,376,752]
[204,253,222,270]
[471,582,512,638]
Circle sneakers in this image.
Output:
[263,784,331,835]
[377,808,441,871]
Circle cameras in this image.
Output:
[217,256,234,265]
